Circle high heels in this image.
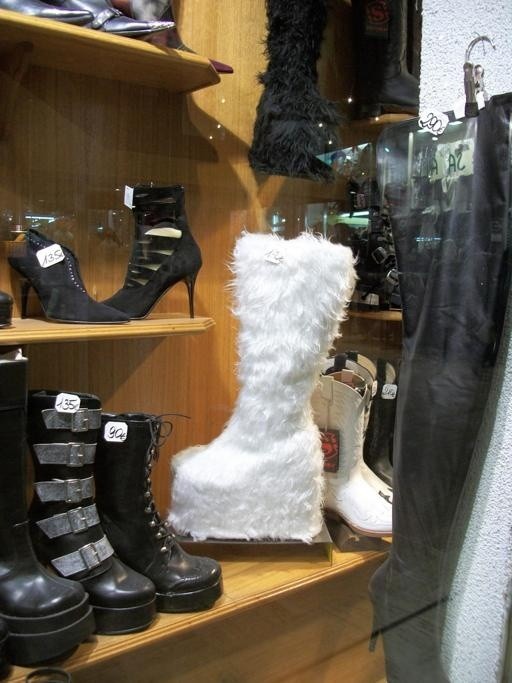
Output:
[2,227,131,325]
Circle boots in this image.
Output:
[102,184,202,319]
[170,234,355,543]
[348,0,421,116]
[369,90,512,677]
[1,348,95,665]
[95,410,223,614]
[311,349,399,537]
[26,389,157,635]
[250,0,339,180]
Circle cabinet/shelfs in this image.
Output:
[1,0,461,683]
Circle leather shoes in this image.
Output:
[0,0,234,74]
